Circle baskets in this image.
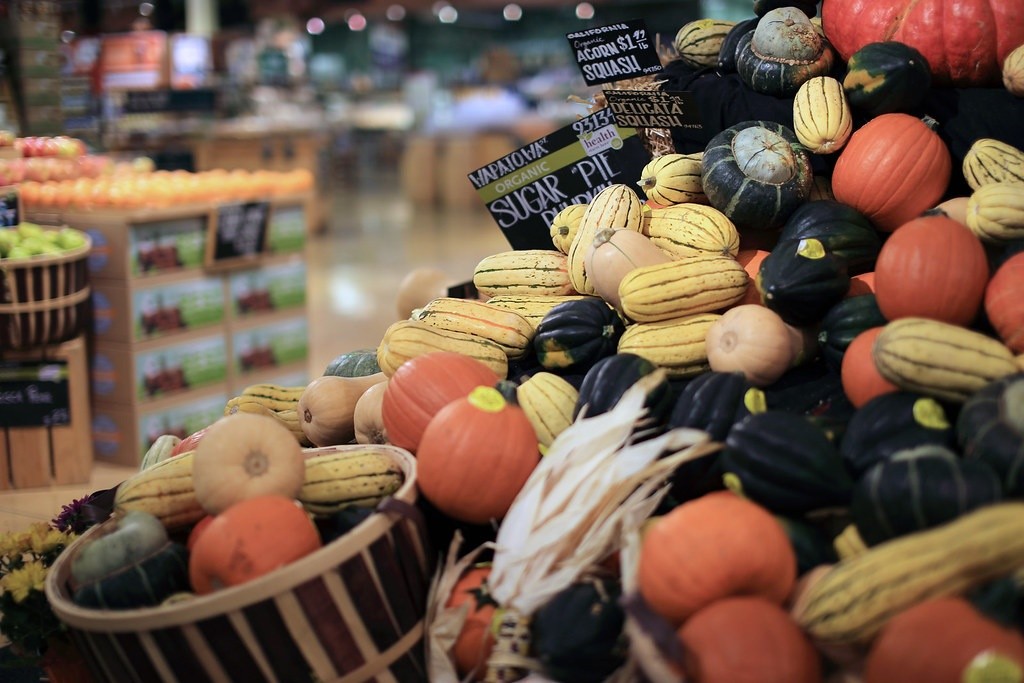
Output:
[43,441,433,682]
[0,222,92,347]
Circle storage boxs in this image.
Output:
[225,253,311,325]
[92,381,230,469]
[9,46,61,78]
[93,324,226,405]
[0,0,62,50]
[22,106,64,134]
[229,362,311,399]
[17,76,62,107]
[226,309,314,385]
[266,191,314,263]
[94,267,227,348]
[24,206,210,281]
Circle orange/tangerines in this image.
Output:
[18,170,312,208]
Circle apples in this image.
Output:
[0,129,152,185]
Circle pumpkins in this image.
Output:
[62,0,1024,683]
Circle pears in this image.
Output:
[0,221,84,261]
